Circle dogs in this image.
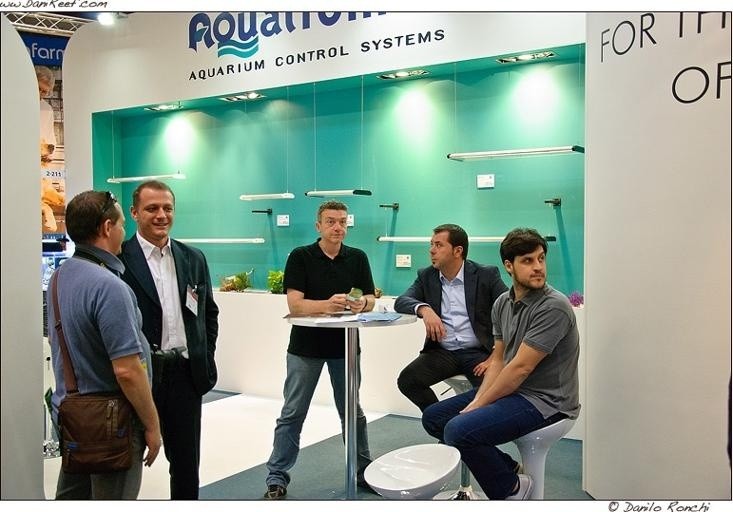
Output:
[40,138,66,233]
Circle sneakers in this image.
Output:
[264,485,287,499]
[505,463,534,500]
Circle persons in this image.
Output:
[34,65,58,163]
[260,199,377,500]
[394,223,510,414]
[421,226,539,500]
[47,189,163,500]
[104,178,220,500]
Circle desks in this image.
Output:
[289,312,417,498]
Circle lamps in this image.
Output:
[377,199,560,242]
[447,45,583,162]
[106,74,371,244]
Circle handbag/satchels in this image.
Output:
[60,397,135,474]
[151,349,194,414]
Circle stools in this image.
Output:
[363,375,575,500]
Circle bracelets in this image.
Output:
[414,302,431,318]
[362,297,368,312]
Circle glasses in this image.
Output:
[96,191,117,231]
